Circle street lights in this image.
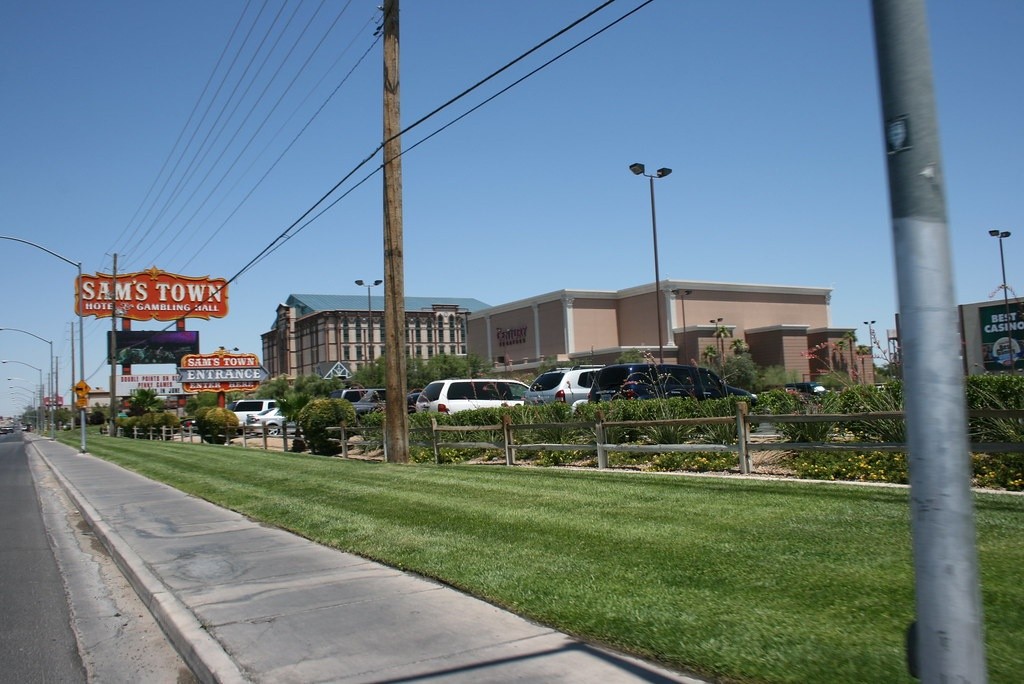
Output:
[864,320,876,351]
[355,279,384,362]
[0,359,43,437]
[6,377,37,433]
[989,230,1020,375]
[0,328,54,441]
[629,162,673,363]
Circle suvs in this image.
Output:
[416,377,531,418]
[588,360,757,409]
[326,388,389,418]
[225,399,287,435]
[524,364,609,412]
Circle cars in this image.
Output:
[788,381,832,396]
[182,418,200,434]
[247,406,305,437]
[1,417,29,434]
[405,392,421,414]
